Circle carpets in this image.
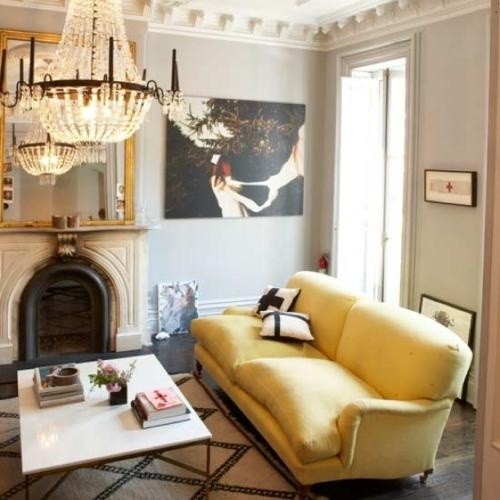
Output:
[1,372,323,499]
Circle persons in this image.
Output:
[210,155,251,217]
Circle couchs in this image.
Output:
[190,271,474,487]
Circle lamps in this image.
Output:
[5,36,79,185]
[0,0,181,164]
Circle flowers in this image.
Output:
[88,357,138,392]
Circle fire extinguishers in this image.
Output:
[319,253,330,273]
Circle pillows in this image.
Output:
[253,285,316,342]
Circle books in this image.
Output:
[131,401,191,430]
[32,362,85,408]
[144,389,181,410]
[136,389,186,419]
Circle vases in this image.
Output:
[110,383,127,405]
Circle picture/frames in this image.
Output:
[417,294,477,406]
[424,168,477,208]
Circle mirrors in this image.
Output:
[0,30,135,227]
